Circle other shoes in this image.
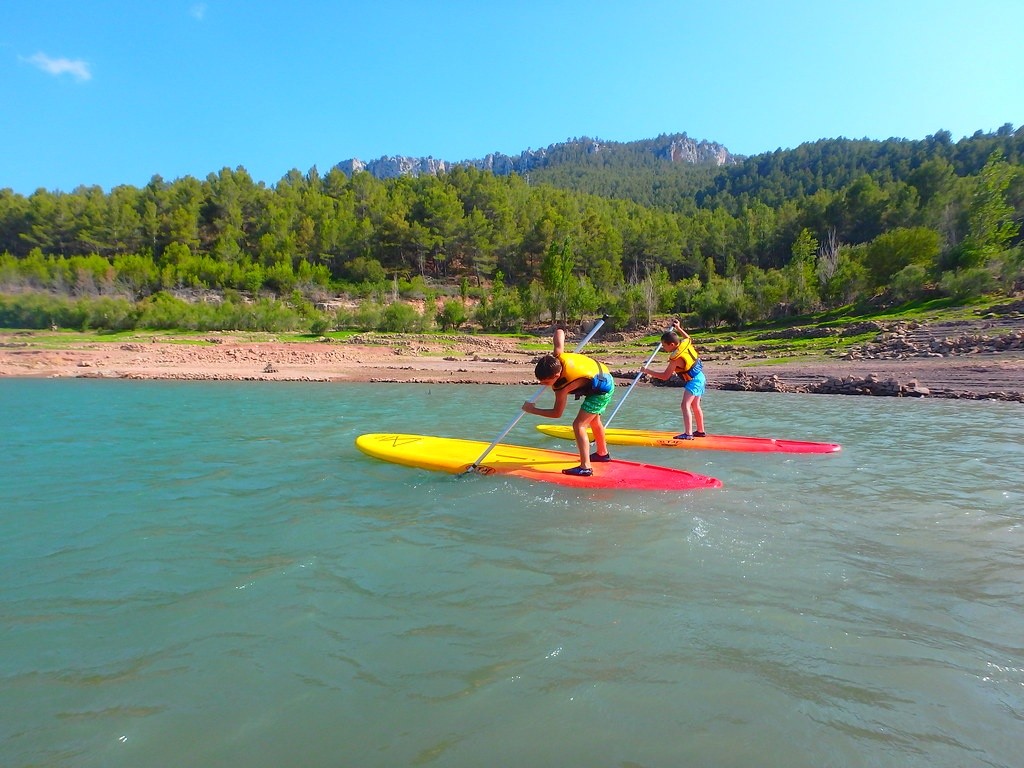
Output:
[673,432,694,440]
[589,451,611,462]
[693,431,706,437]
[562,466,593,477]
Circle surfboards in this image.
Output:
[354,432,722,493]
[535,423,841,454]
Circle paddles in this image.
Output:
[593,319,678,444]
[453,314,610,482]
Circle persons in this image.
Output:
[639,320,708,441]
[521,328,615,477]
